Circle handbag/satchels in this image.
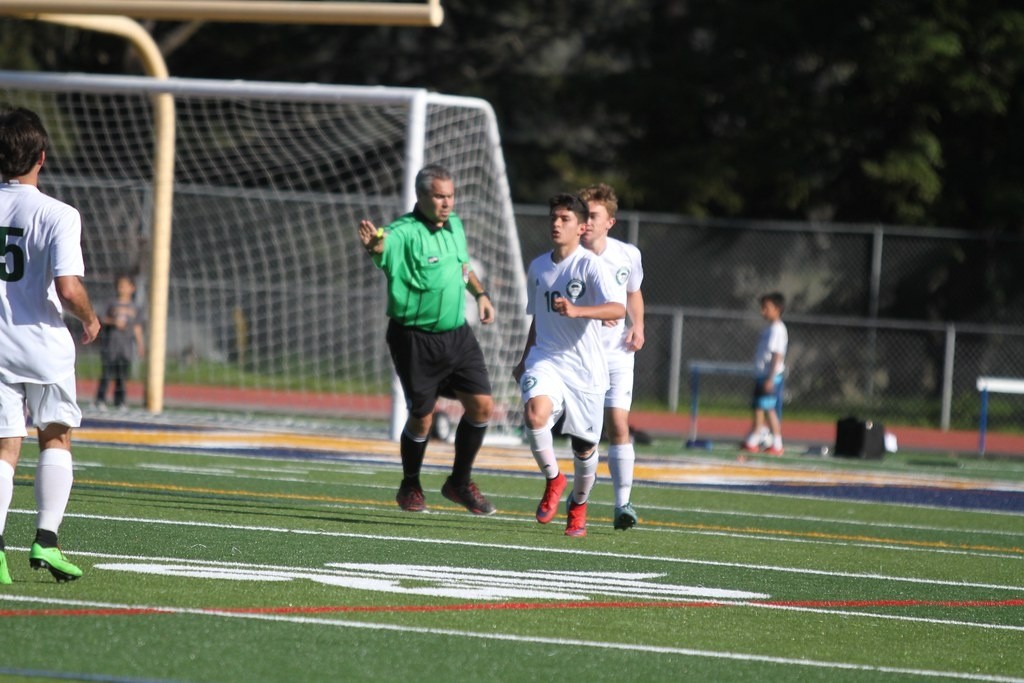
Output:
[834,417,884,461]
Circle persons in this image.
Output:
[0,104,102,584]
[95,272,143,412]
[578,181,644,528]
[743,292,790,458]
[361,166,499,517]
[512,192,625,537]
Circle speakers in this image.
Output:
[835,416,885,460]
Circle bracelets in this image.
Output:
[476,291,490,301]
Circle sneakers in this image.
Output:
[740,440,760,453]
[565,490,588,537]
[763,445,786,456]
[441,474,497,515]
[536,473,568,523]
[614,502,638,530]
[0,550,15,585]
[28,543,84,582]
[396,479,425,511]
